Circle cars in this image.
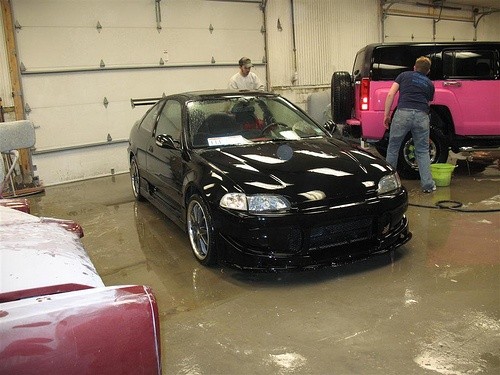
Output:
[1,200,163,375]
[127,89,413,273]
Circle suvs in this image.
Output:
[330,41,500,181]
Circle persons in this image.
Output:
[383,56,436,192]
[230,57,264,91]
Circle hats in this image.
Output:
[239,56,254,68]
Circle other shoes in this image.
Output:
[423,185,437,193]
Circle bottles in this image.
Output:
[294,72,299,86]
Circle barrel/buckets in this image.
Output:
[430,163,458,186]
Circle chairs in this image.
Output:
[189,103,258,146]
